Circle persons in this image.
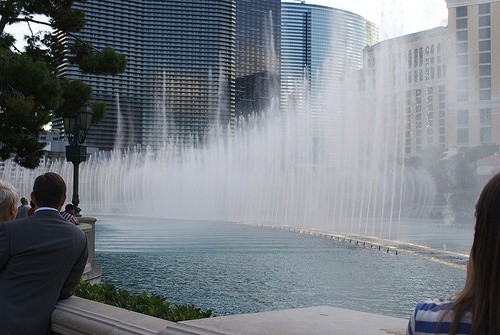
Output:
[406,173,500,335]
[0,172,88,335]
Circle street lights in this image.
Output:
[62,104,94,217]
